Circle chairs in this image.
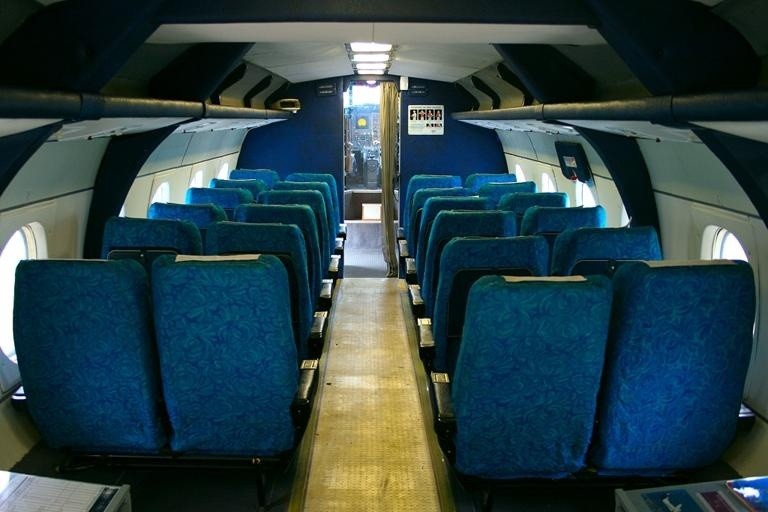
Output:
[0,166,351,511]
[388,169,767,510]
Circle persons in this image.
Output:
[411,110,441,120]
